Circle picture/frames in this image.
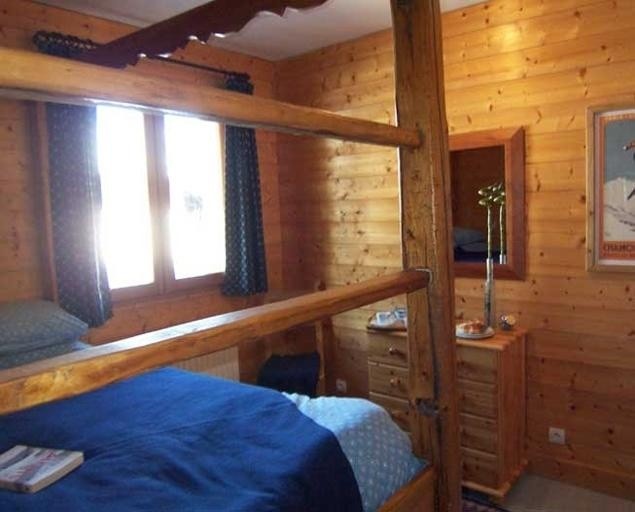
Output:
[583,97,635,274]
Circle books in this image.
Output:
[0,442,86,494]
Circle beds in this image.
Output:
[1,266,461,511]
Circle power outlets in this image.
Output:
[548,427,567,445]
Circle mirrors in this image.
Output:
[448,125,528,279]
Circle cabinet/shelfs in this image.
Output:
[365,307,531,504]
[261,280,328,398]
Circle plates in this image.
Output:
[455,323,495,340]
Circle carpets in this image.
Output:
[453,494,517,511]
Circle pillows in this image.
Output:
[2,296,89,372]
[450,223,482,250]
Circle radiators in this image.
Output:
[161,339,238,383]
